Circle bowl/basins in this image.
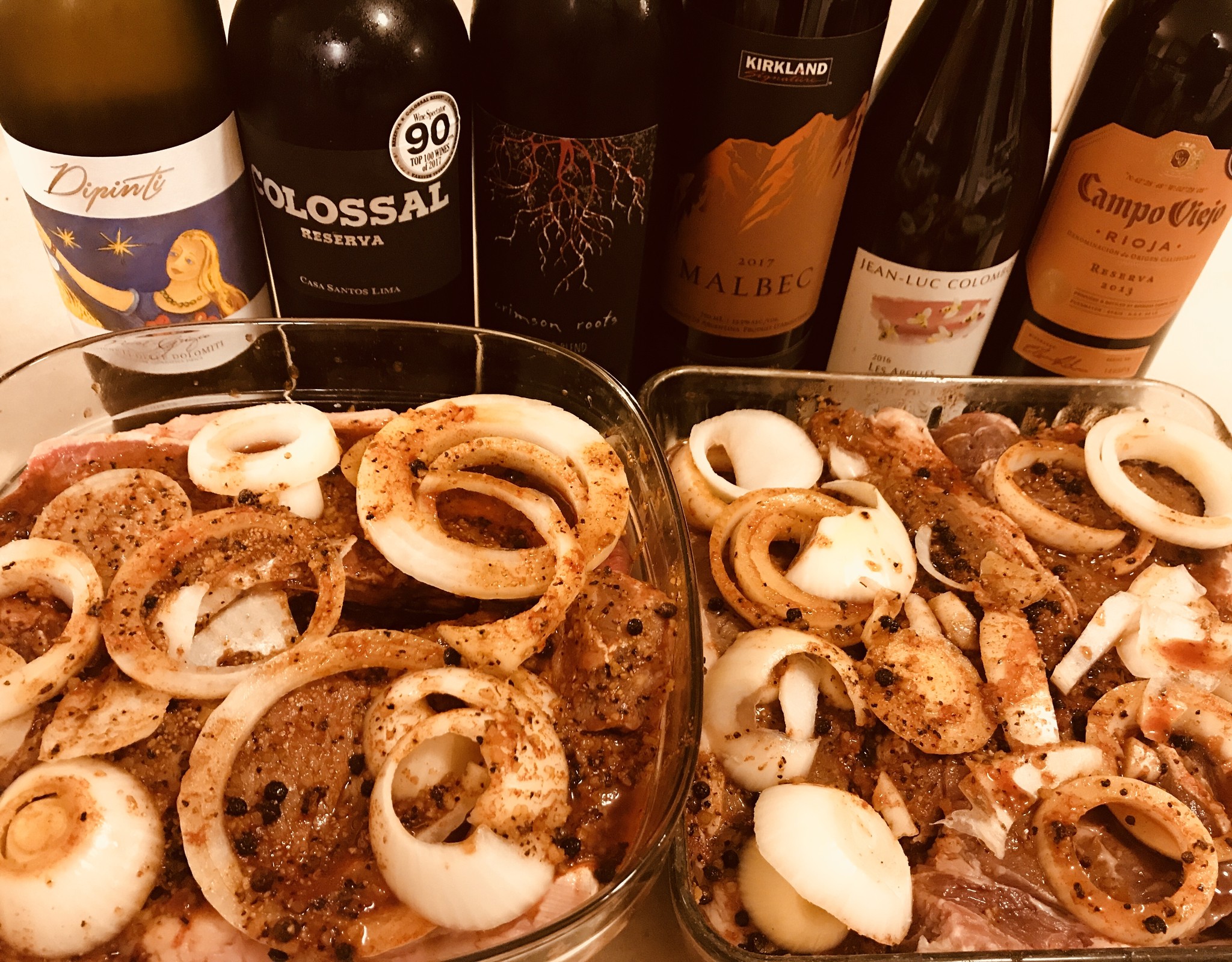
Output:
[0,318,704,962]
[635,366,1232,962]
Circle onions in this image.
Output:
[1,391,632,962]
[687,402,1232,954]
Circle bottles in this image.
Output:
[673,0,893,431]
[973,0,1232,428]
[810,0,1056,428]
[469,0,684,421]
[225,0,471,412]
[0,0,289,430]
